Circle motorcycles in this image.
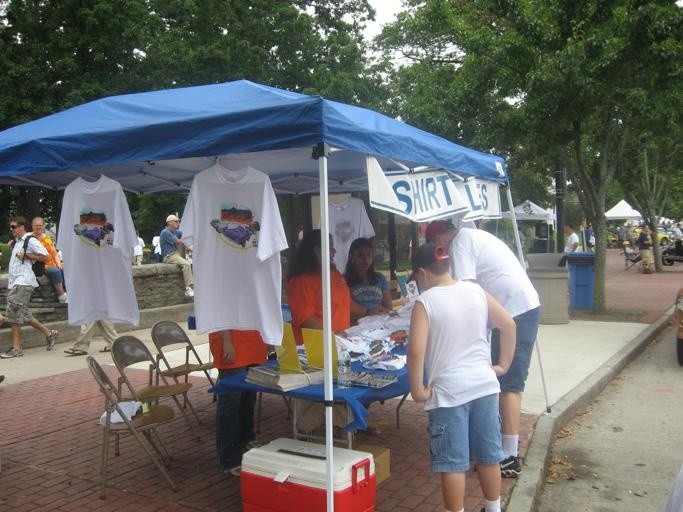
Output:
[659,240,682,267]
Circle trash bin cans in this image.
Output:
[526,252,569,324]
[566,253,596,308]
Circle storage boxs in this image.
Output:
[238,438,376,511]
[334,442,390,489]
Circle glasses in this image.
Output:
[9,224,21,229]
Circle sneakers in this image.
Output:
[0,344,24,358]
[46,329,60,352]
[58,292,69,304]
[498,454,523,478]
[184,287,195,297]
[219,464,241,477]
[241,440,262,454]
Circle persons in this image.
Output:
[286,228,351,342]
[207,330,269,478]
[513,220,683,274]
[343,237,394,326]
[405,243,516,512]
[0,214,194,360]
[425,219,541,476]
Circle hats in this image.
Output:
[165,214,180,223]
[424,220,454,242]
[405,242,451,285]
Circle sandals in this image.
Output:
[63,348,88,356]
[99,346,111,353]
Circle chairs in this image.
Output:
[111,335,198,458]
[622,245,641,271]
[150,321,219,408]
[84,356,180,500]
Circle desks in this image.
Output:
[207,305,426,452]
[186,305,293,402]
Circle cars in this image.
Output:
[656,228,670,246]
[674,287,682,367]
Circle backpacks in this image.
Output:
[11,235,45,277]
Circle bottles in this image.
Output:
[337,346,351,389]
